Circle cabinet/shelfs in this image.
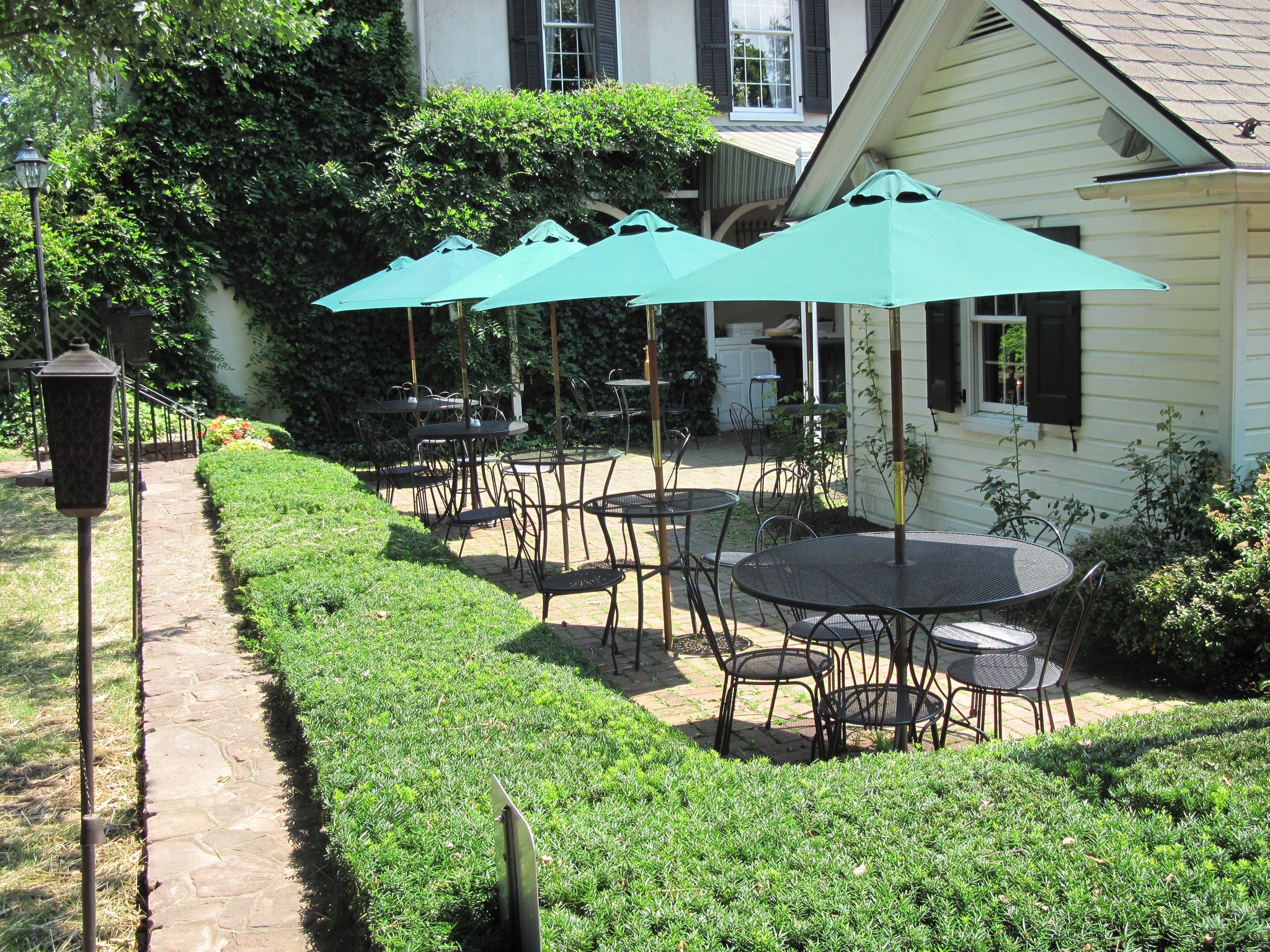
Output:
[703,338,777,432]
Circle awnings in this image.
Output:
[697,124,827,212]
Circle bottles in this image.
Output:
[408,397,418,404]
[470,418,481,427]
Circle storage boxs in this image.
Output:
[817,321,834,333]
[725,322,764,338]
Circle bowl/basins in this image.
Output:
[766,328,798,339]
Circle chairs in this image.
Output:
[359,367,1108,766]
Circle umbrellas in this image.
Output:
[339,235,500,510]
[625,170,1170,753]
[470,210,742,652]
[311,256,456,466]
[420,220,588,571]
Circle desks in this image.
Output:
[732,530,1073,751]
[604,378,672,455]
[408,420,528,525]
[500,446,622,586]
[583,489,740,672]
[357,398,478,467]
[766,404,846,497]
[748,377,783,443]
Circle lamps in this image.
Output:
[95,293,113,326]
[31,335,123,518]
[117,298,154,368]
[107,293,128,347]
